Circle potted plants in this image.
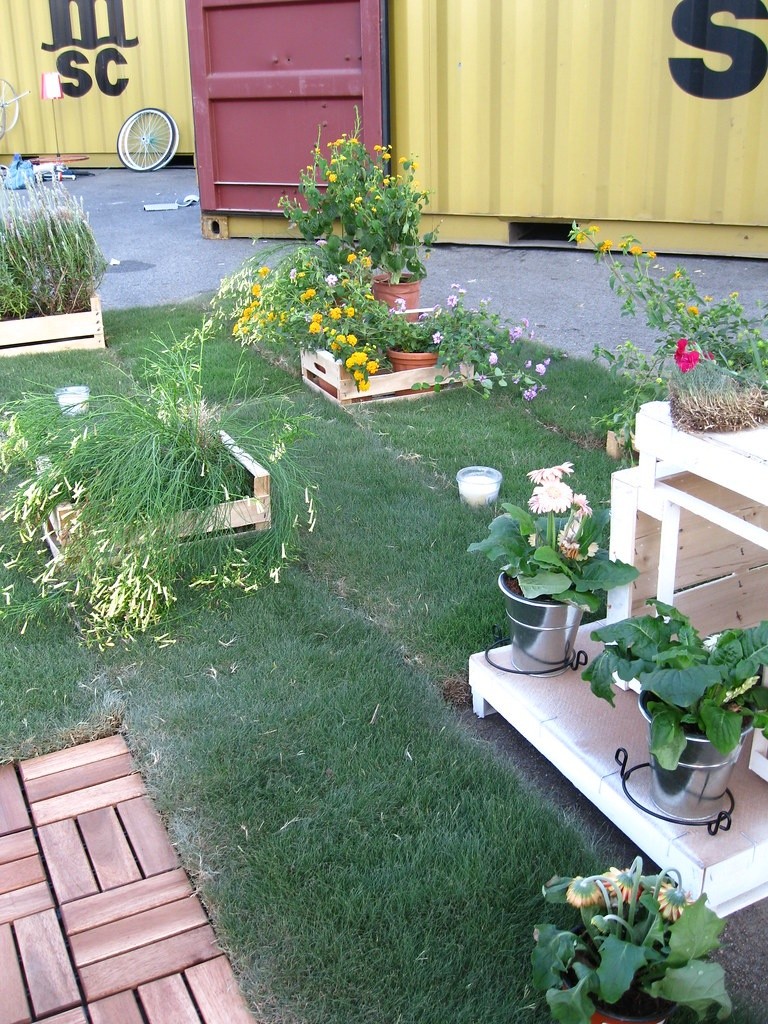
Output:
[211,103,554,409]
[468,461,641,678]
[582,594,768,820]
